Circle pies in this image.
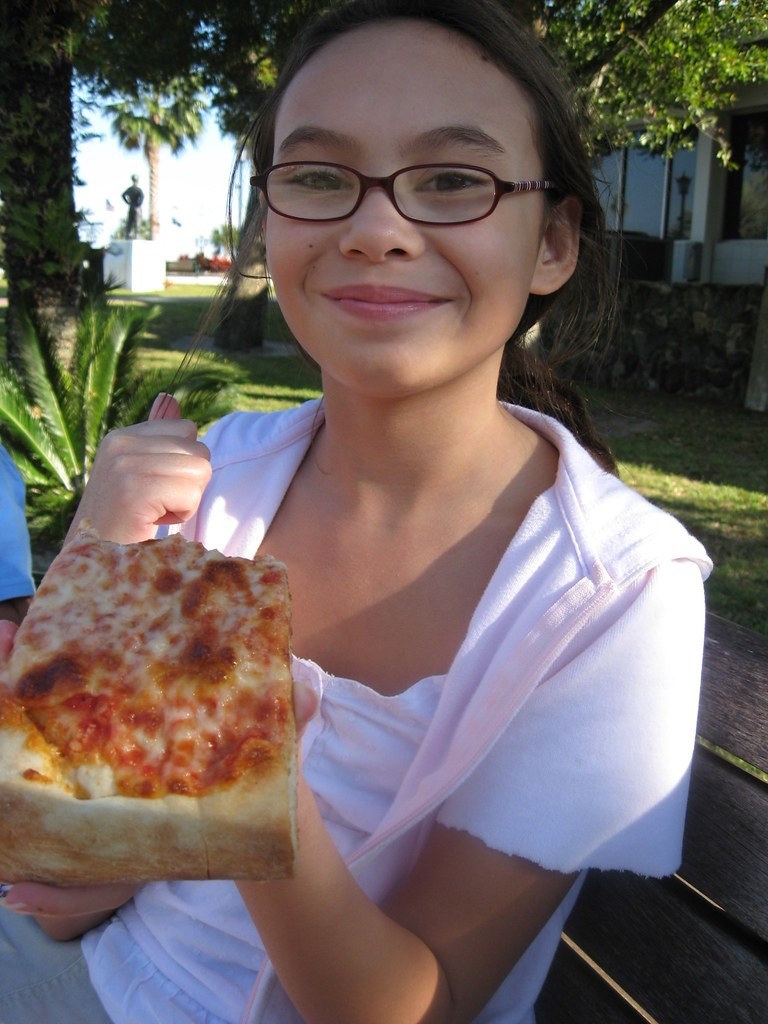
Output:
[0,520,298,881]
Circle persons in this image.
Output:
[123,173,145,239]
[0,0,714,1024]
[0,441,37,667]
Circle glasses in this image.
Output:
[249,161,566,224]
